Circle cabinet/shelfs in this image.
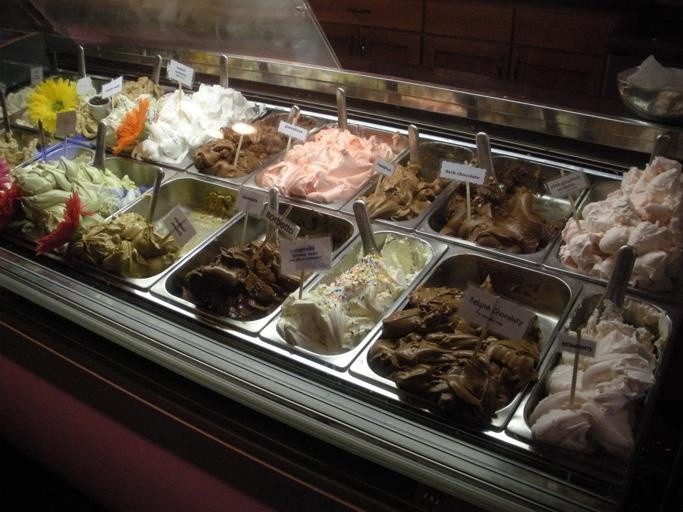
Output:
[1,0,683,512]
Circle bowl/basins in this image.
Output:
[615,66,683,125]
